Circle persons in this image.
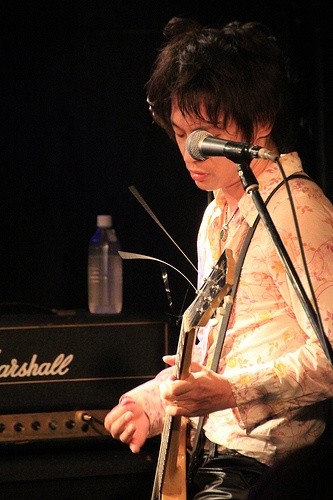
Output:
[104,17,333,499]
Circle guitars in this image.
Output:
[148,247,240,496]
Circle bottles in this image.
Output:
[88,214,122,315]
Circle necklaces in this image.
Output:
[219,201,239,242]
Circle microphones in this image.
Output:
[185,129,279,162]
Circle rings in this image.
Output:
[172,400,179,406]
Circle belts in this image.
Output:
[185,430,232,452]
